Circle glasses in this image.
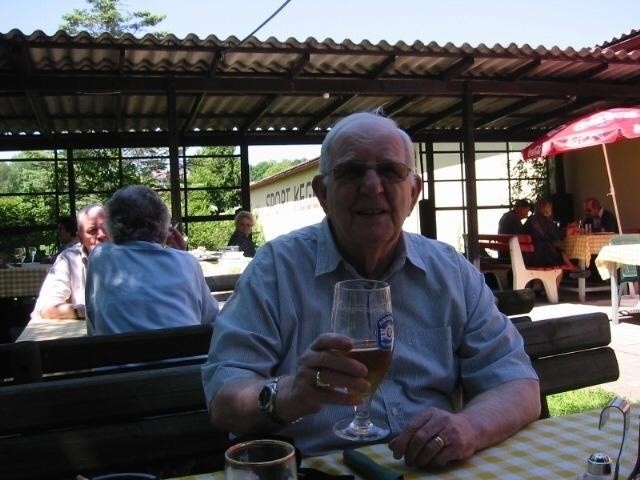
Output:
[323,159,413,184]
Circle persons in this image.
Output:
[498,199,530,264]
[31,205,112,321]
[39,215,80,264]
[524,201,577,272]
[201,112,541,468]
[226,212,257,258]
[582,199,620,233]
[84,184,219,335]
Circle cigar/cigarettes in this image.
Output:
[168,222,180,236]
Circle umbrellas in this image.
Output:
[521,107,640,234]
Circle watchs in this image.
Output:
[257,376,304,427]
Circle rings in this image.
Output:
[314,370,329,390]
[434,435,445,450]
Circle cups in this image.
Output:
[331,279,393,443]
[22,248,26,264]
[585,223,591,236]
[29,247,37,265]
[225,440,296,479]
[15,248,22,264]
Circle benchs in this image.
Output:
[1,313,621,480]
[466,235,563,304]
[1,287,538,379]
